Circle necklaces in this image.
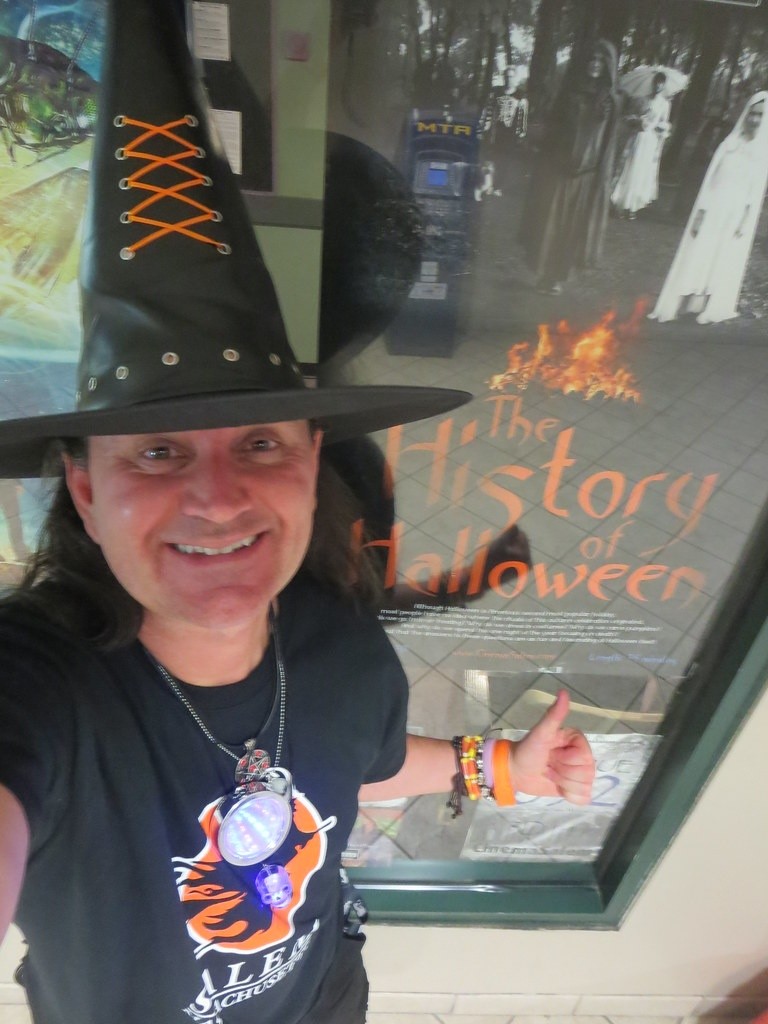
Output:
[146,600,294,908]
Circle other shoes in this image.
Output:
[616,208,625,219]
[628,210,635,219]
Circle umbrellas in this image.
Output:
[619,65,688,99]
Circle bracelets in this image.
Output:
[446,734,516,819]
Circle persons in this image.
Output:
[475,33,671,295]
[410,39,459,122]
[645,91,767,324]
[0,416,595,1024]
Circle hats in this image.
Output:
[0,0,427,479]
[184,0,423,379]
